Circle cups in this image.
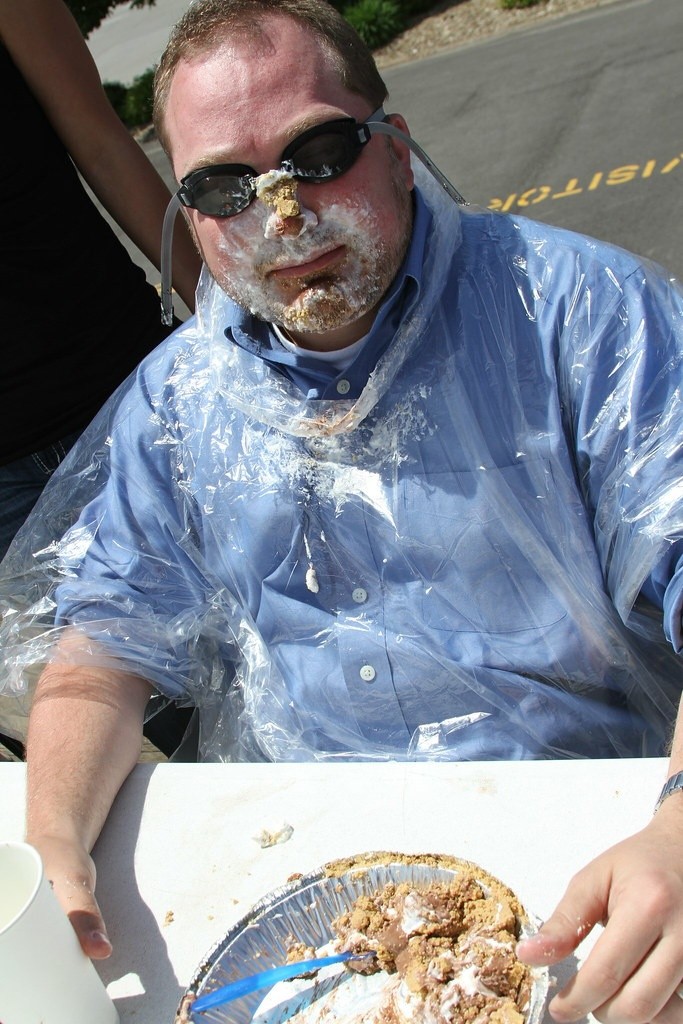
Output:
[0,840,121,1024]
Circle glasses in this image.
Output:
[177,109,387,218]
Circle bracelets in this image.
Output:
[653,770,683,815]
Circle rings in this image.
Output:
[675,982,683,999]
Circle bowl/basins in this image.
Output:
[175,851,551,1024]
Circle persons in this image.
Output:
[0,0,203,564]
[0,0,683,1024]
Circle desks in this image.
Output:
[1,755,670,1022]
